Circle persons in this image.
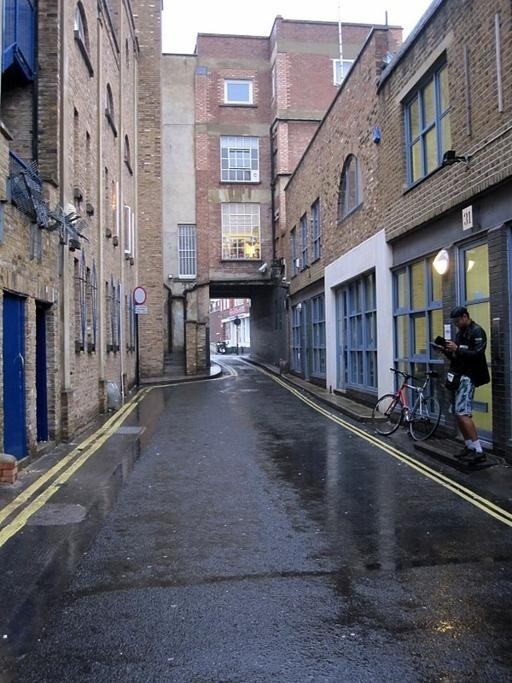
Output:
[443,306,490,463]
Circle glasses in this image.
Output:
[433,249,450,274]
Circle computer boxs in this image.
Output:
[258,262,267,273]
[443,150,471,171]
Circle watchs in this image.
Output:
[216,340,229,353]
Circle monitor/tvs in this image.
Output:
[428,335,447,351]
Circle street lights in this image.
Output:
[445,369,459,390]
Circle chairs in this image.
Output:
[460,448,487,463]
[453,446,473,457]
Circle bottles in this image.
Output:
[449,305,467,322]
